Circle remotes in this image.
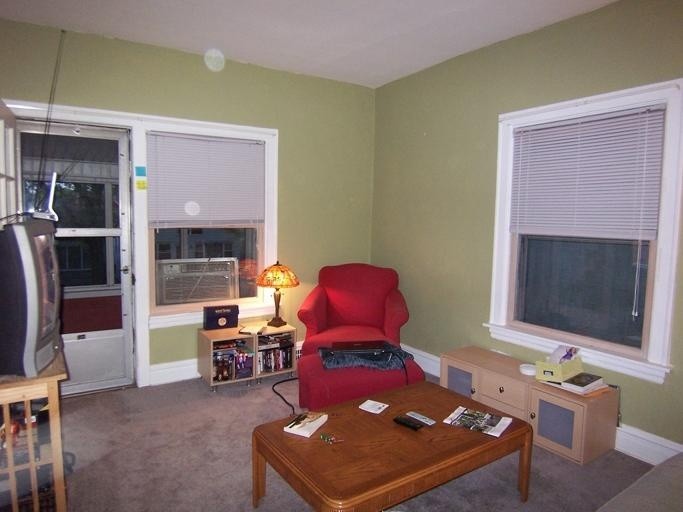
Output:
[405,411,436,426]
[393,416,423,431]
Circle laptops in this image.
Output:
[332,340,385,357]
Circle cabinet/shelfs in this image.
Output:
[439,346,619,467]
[198,319,296,387]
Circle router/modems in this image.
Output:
[33,172,59,222]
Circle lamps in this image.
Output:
[256,261,299,327]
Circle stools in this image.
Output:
[297,353,425,411]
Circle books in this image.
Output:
[560,373,605,392]
[213,332,293,381]
[358,400,389,415]
[282,410,329,438]
[442,405,512,438]
[238,324,267,335]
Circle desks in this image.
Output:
[0,350,69,512]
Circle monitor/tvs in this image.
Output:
[0,219,64,378]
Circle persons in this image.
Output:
[546,344,580,364]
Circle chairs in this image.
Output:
[297,262,409,357]
